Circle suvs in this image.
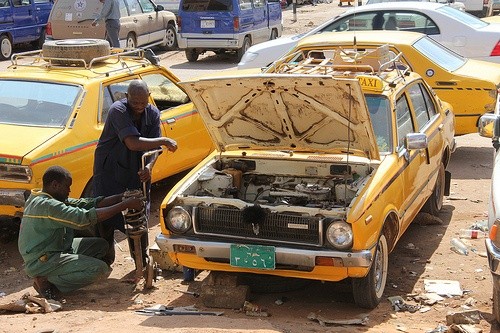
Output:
[365,1,499,17]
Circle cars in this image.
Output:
[153,0,180,25]
[265,31,500,137]
[279,0,320,8]
[158,38,455,311]
[0,38,217,218]
[478,86,499,326]
[43,0,178,57]
[238,3,500,67]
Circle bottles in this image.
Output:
[450,237,469,255]
[458,229,488,239]
[471,246,487,257]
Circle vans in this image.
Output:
[176,0,282,62]
[0,0,52,61]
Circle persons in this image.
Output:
[91,0,121,53]
[18,166,145,300]
[92,79,177,269]
[371,15,385,30]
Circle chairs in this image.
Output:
[385,16,397,30]
